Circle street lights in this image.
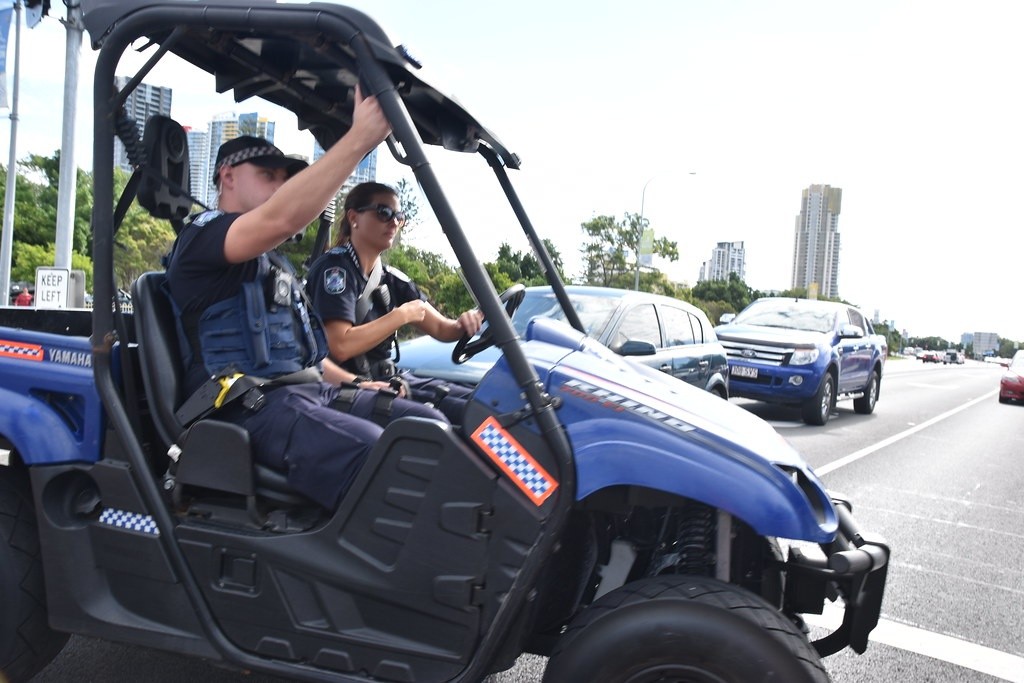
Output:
[634,172,697,289]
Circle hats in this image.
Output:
[212,135,309,185]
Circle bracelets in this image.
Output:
[352,376,372,385]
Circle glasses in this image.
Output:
[356,202,403,223]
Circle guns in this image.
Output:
[236,387,267,414]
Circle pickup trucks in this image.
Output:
[714,296,888,426]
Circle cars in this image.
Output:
[903,346,965,365]
[388,284,731,401]
[998,349,1024,404]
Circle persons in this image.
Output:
[305,181,482,426]
[167,86,451,515]
[15,288,33,306]
